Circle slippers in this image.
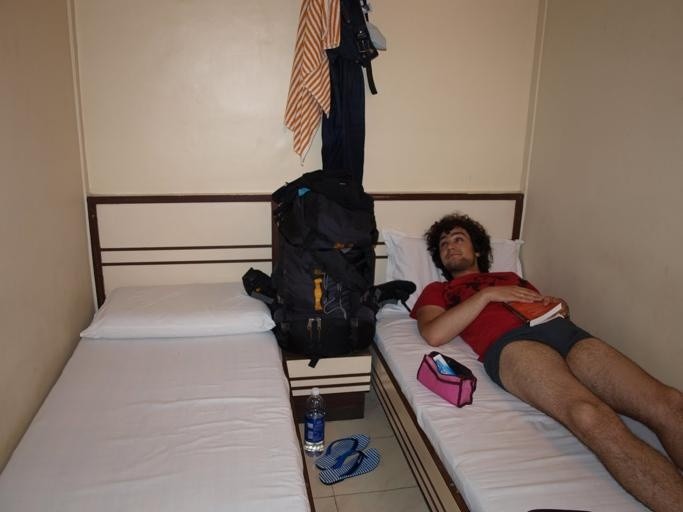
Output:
[314,432,369,470]
[318,445,380,486]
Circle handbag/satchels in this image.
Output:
[416,348,479,409]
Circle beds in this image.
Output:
[0,193,317,511]
[361,190,681,512]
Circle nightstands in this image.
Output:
[282,343,371,424]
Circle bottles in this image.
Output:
[302,387,324,457]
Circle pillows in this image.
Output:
[77,285,276,338]
[380,228,525,315]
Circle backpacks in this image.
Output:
[270,169,380,359]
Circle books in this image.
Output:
[502,295,564,329]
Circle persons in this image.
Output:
[407,214,683,510]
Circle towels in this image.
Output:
[283,0,342,168]
